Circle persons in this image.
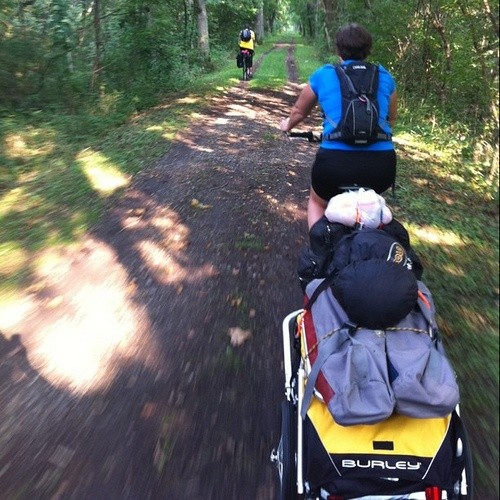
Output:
[238,24,255,79]
[277,25,399,235]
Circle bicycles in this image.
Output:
[241,29,249,81]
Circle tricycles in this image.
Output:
[270,131,473,500]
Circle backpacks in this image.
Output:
[241,29,251,41]
[329,63,388,148]
